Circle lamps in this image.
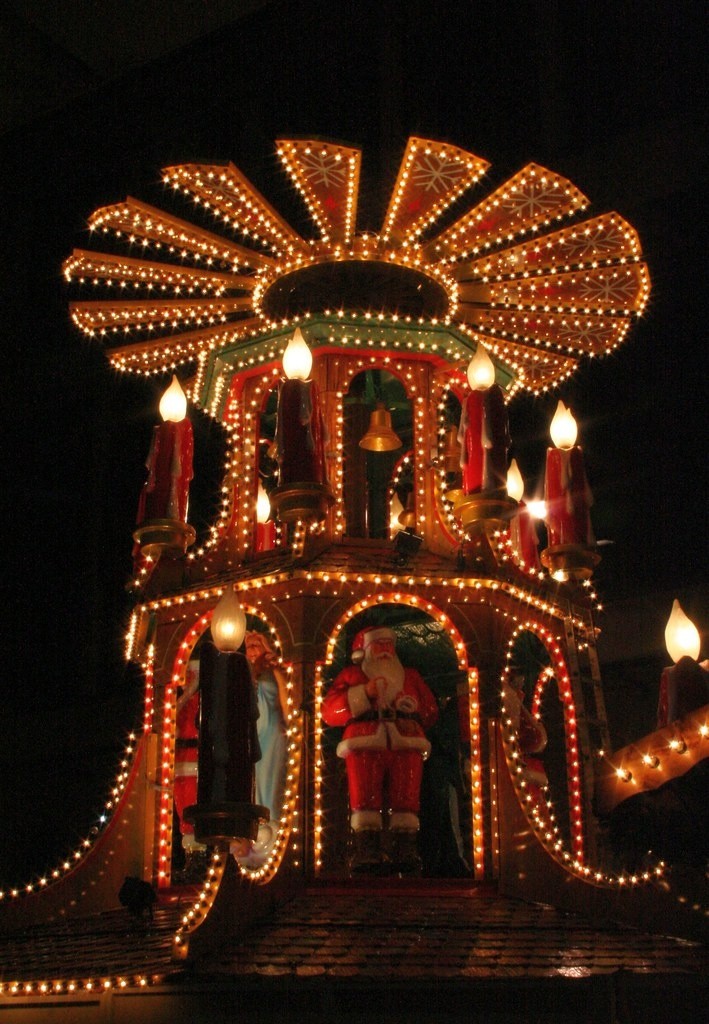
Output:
[505,458,540,578]
[656,593,709,870]
[179,578,271,851]
[452,341,516,547]
[131,374,198,557]
[256,482,274,556]
[540,401,601,583]
[266,328,335,524]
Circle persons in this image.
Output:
[165,630,288,886]
[504,658,571,884]
[320,625,439,877]
